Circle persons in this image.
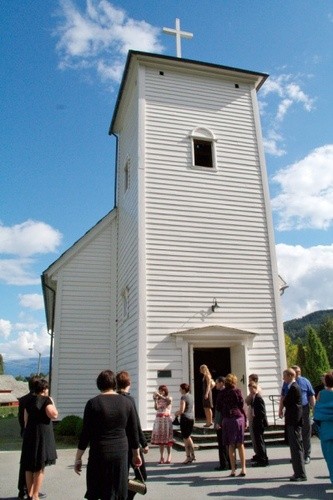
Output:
[200,364,214,426]
[74,370,150,500]
[213,374,247,477]
[313,371,333,487]
[279,365,316,481]
[245,374,269,467]
[151,385,174,464]
[174,383,195,465]
[18,376,57,500]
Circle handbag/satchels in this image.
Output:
[128,466,147,495]
[172,416,179,426]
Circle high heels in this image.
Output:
[183,458,196,465]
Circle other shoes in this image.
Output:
[304,457,310,464]
[166,458,172,464]
[215,464,237,471]
[290,474,307,481]
[160,457,165,463]
[251,458,268,467]
[18,491,47,500]
